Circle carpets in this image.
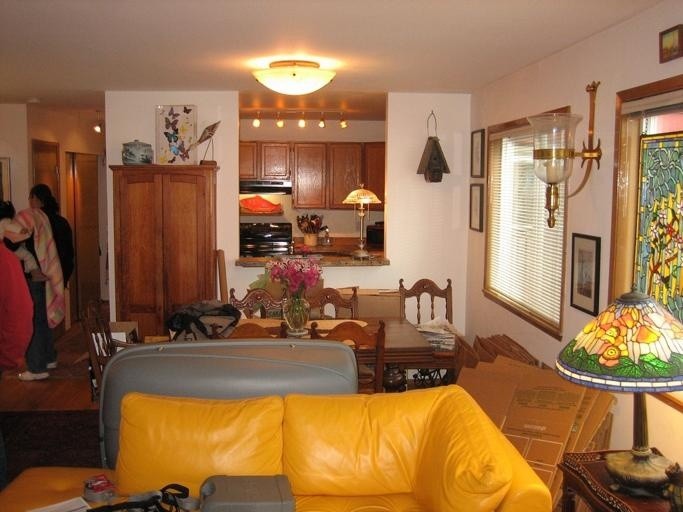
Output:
[0,409,103,492]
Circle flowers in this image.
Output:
[265,253,323,329]
[295,214,327,233]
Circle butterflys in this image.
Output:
[163,107,185,155]
[182,106,192,115]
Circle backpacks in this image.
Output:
[165,299,241,341]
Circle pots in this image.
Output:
[121,139,155,165]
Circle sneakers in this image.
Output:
[48,363,57,371]
[20,369,49,381]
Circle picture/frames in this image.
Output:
[568,231,602,317]
[468,128,486,233]
[657,23,683,65]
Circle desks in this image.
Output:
[554,445,683,512]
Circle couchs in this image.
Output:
[1,383,554,511]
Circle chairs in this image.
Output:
[76,298,126,403]
[209,276,464,386]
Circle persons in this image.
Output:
[0,199,51,282]
[0,183,74,382]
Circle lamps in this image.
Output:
[250,60,336,97]
[250,109,348,130]
[340,183,382,259]
[553,284,681,502]
[524,79,603,229]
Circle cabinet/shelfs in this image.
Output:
[237,139,384,210]
[108,163,219,344]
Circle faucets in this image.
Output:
[288,240,295,255]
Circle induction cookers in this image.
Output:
[240,238,294,258]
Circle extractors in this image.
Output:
[240,179,292,196]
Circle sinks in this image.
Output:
[293,250,346,259]
